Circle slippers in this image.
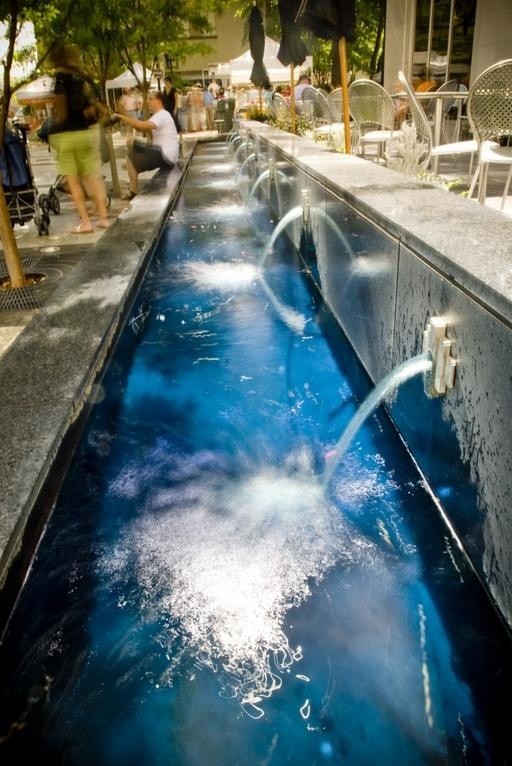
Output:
[121,190,137,200]
[95,220,110,229]
[71,225,94,234]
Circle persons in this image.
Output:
[108,91,179,201]
[116,70,323,133]
[47,45,112,234]
[93,97,110,163]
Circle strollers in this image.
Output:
[38,110,120,215]
[0,122,50,236]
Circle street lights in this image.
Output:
[163,53,178,88]
